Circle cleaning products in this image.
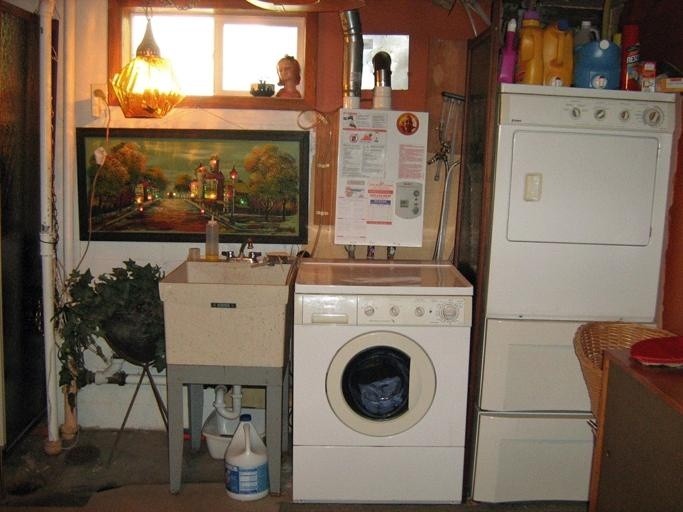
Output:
[498,12,622,90]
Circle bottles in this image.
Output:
[204,215,219,261]
[621,25,639,91]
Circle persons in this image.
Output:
[276,55,301,98]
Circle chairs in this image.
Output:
[573,320,680,438]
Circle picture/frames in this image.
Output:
[74,126,309,245]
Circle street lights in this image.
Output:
[229,165,238,216]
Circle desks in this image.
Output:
[589,348,683,512]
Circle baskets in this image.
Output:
[573,322,678,419]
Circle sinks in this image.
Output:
[157,259,294,368]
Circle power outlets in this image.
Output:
[91,84,110,118]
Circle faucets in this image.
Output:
[240,238,253,257]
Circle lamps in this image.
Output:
[112,3,186,119]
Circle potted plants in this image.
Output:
[59,259,166,413]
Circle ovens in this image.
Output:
[481,82,678,319]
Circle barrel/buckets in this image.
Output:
[224,415,269,501]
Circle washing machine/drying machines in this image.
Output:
[292,258,474,505]
